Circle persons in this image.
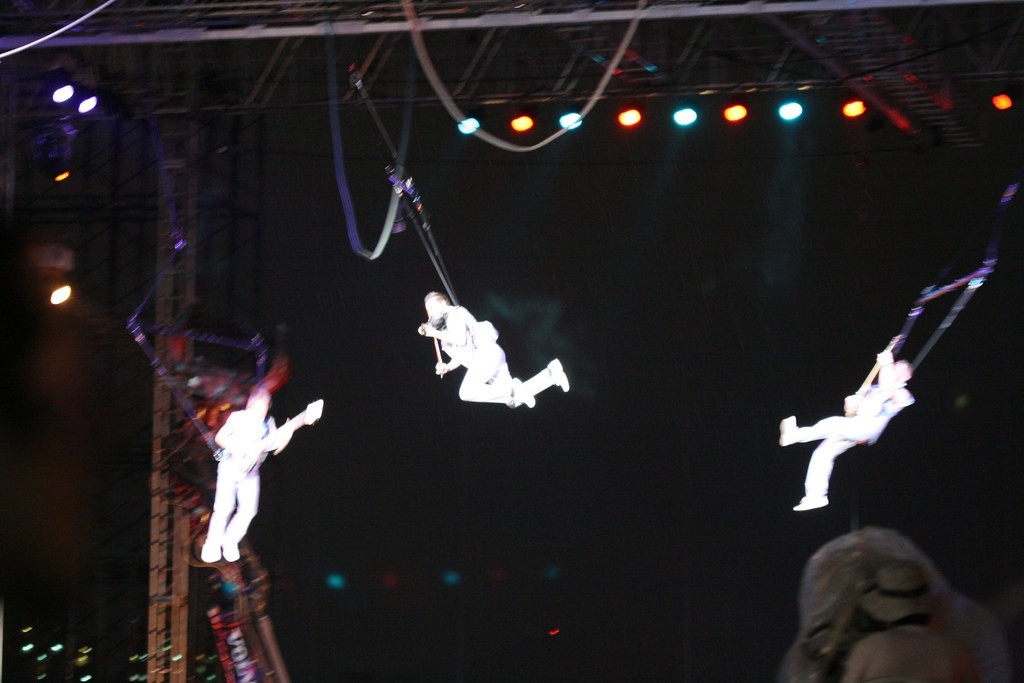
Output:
[842,561,980,682]
[419,291,570,408]
[779,350,915,511]
[200,389,290,563]
